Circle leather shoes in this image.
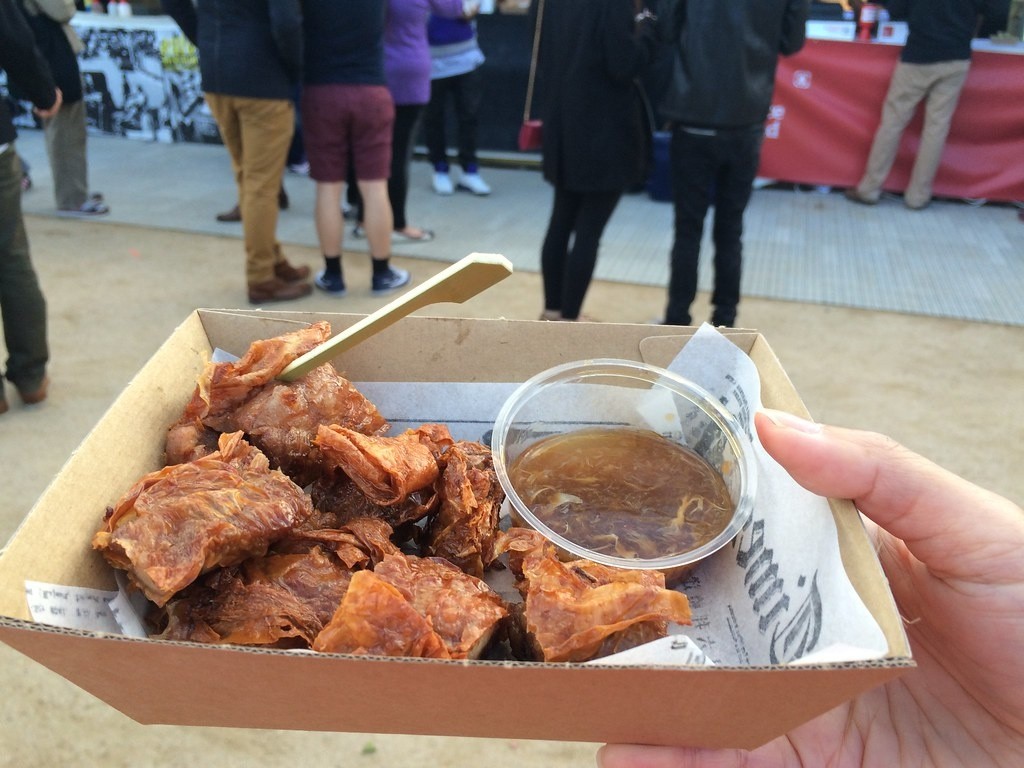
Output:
[273,258,312,280]
[248,278,312,305]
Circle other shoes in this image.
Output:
[457,168,492,197]
[215,205,242,222]
[842,187,881,205]
[16,375,50,405]
[60,192,109,216]
[388,228,434,243]
[428,170,454,195]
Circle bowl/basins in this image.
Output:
[492,356,760,573]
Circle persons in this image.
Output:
[596,408,1024,768]
[28,0,109,217]
[0,0,62,415]
[845,0,1012,210]
[651,0,805,328]
[283,0,411,297]
[163,0,314,303]
[215,0,494,244]
[521,0,677,322]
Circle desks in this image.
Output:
[0,9,1024,213]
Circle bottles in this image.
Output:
[85,0,132,17]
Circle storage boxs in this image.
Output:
[1,310,917,754]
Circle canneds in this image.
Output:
[852,2,879,42]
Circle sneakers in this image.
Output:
[315,268,345,295]
[372,268,411,297]
